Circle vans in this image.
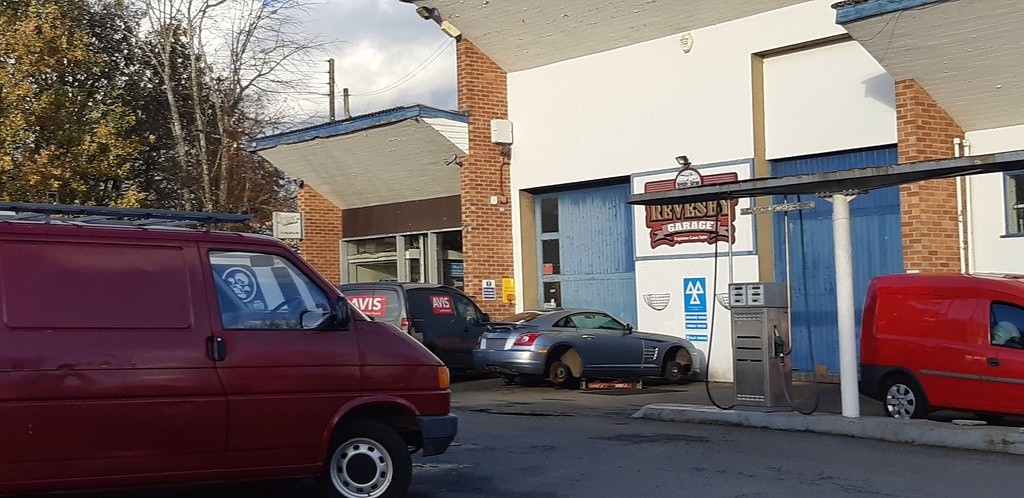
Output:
[335,283,492,375]
[860,273,1023,420]
[1,203,458,498]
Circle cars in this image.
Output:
[473,308,701,385]
